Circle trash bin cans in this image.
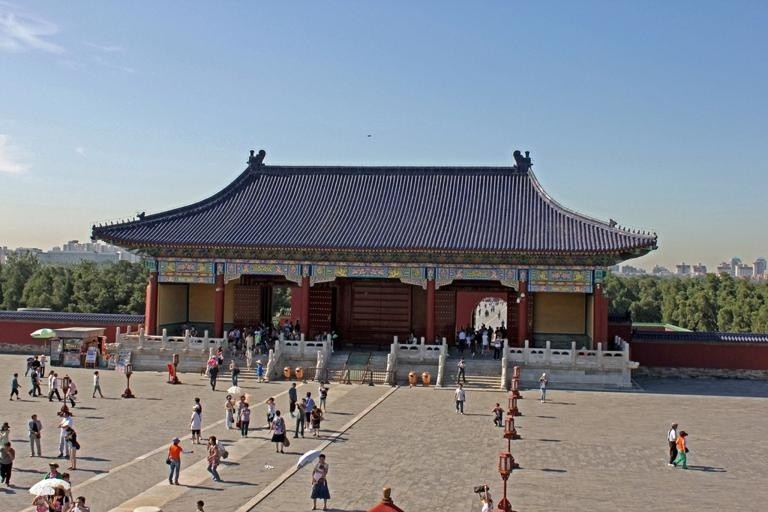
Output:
[422,372,430,387]
[284,366,292,380]
[295,367,304,381]
[408,371,417,386]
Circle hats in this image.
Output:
[171,437,180,443]
[255,359,263,364]
[52,483,66,493]
[3,422,11,428]
[48,462,60,468]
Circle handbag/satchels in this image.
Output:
[284,436,290,447]
[232,408,236,413]
[75,441,81,449]
[165,458,171,465]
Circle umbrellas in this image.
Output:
[28,327,57,352]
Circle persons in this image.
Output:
[478,483,493,510]
[188,317,331,484]
[195,499,205,510]
[674,431,690,469]
[1,352,90,512]
[538,372,549,401]
[435,295,508,359]
[453,383,465,413]
[492,402,505,426]
[166,437,193,484]
[307,454,331,510]
[457,356,468,383]
[667,423,677,465]
[92,370,104,398]
